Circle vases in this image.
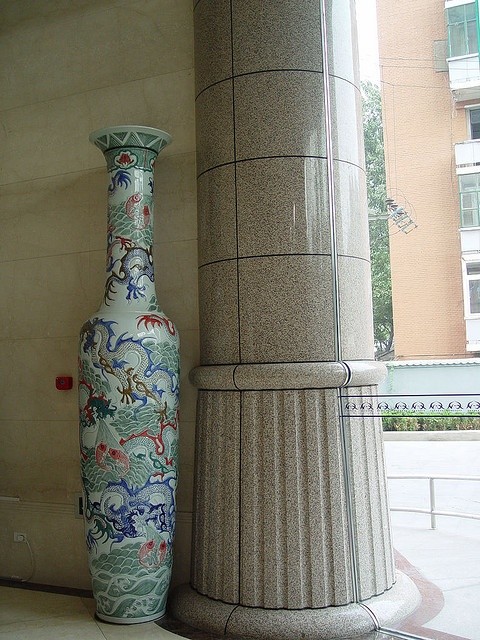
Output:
[75,123,182,624]
